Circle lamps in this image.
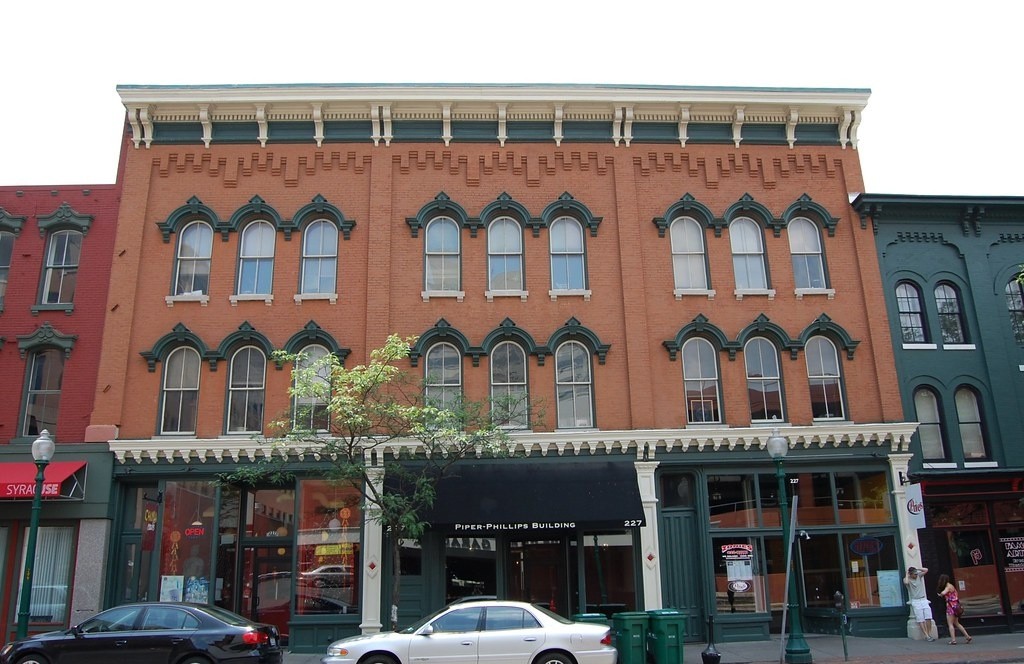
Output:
[328,486,341,532]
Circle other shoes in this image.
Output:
[966,636,972,643]
[924,635,935,641]
[948,640,956,645]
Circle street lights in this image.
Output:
[15,428,56,642]
[768,427,814,664]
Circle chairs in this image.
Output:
[164,612,178,629]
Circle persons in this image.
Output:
[903,567,936,642]
[937,574,972,645]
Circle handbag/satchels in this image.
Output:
[953,605,963,616]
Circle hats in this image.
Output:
[910,569,918,574]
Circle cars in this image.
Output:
[299,565,354,588]
[246,574,356,614]
[319,597,619,664]
[0,598,283,664]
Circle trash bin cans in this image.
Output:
[646,609,686,664]
[571,613,608,625]
[612,612,651,664]
